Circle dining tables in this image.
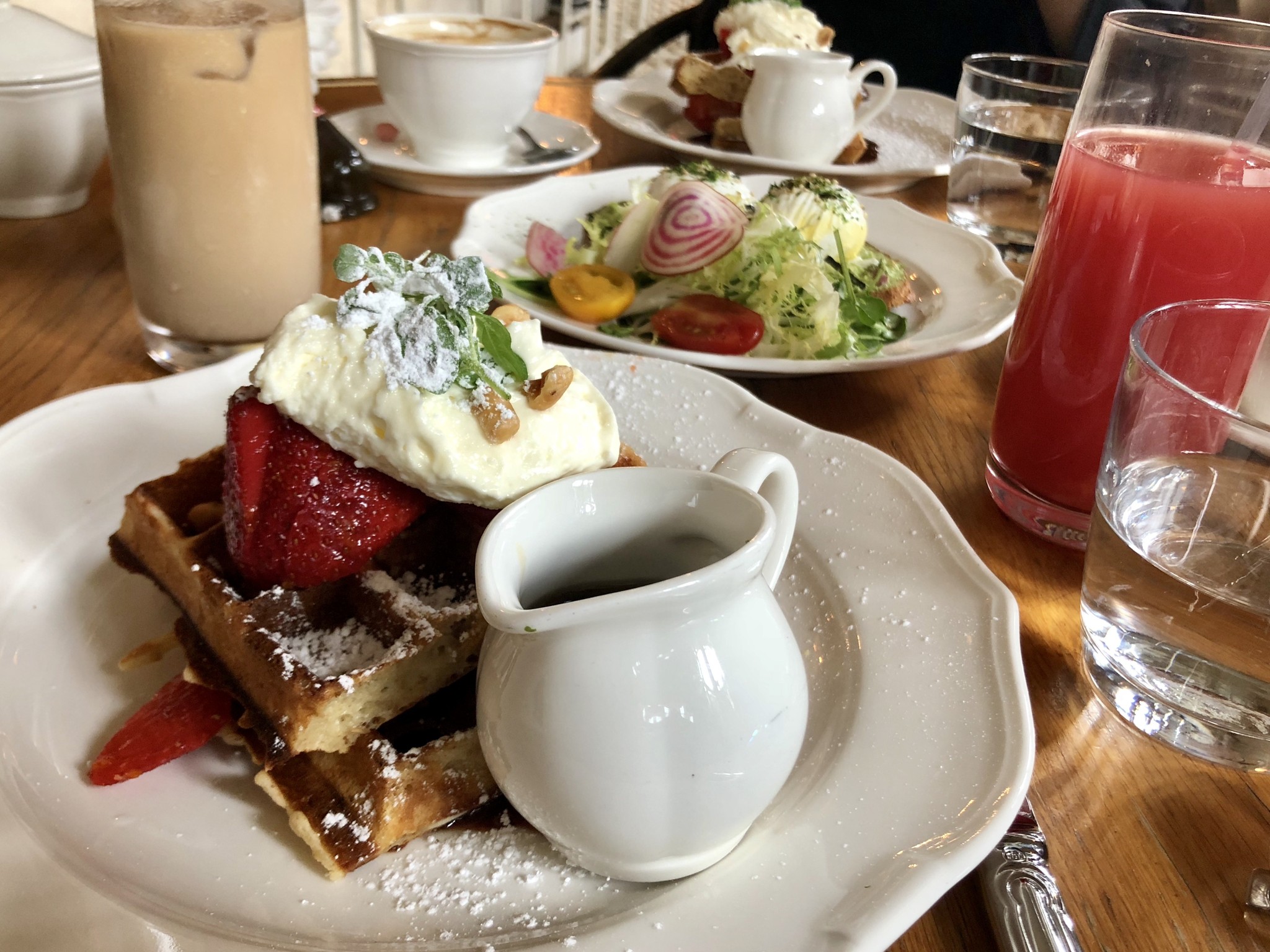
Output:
[1,71,1269,952]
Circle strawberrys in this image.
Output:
[218,388,432,593]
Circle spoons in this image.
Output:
[516,127,575,165]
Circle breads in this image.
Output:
[101,449,503,878]
[667,48,868,168]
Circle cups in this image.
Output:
[945,52,1090,266]
[361,14,559,171]
[1080,297,1270,774]
[0,63,107,217]
[984,5,1270,552]
[94,0,321,375]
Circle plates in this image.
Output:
[0,347,1034,950]
[451,163,1027,375]
[591,78,974,196]
[329,106,602,198]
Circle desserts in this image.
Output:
[646,159,867,262]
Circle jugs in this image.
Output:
[472,448,807,890]
[741,46,897,165]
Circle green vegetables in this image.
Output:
[330,242,531,402]
[482,187,908,362]
